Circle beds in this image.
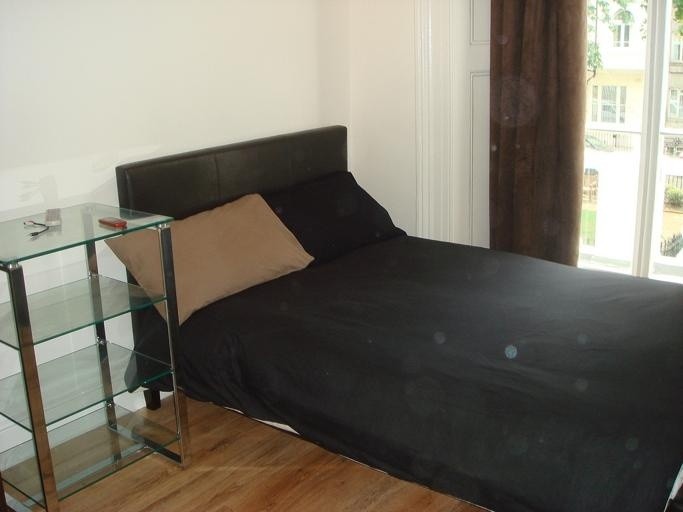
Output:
[114,122,680,512]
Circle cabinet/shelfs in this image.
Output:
[0,204,192,512]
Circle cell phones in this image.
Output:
[99,216,127,227]
